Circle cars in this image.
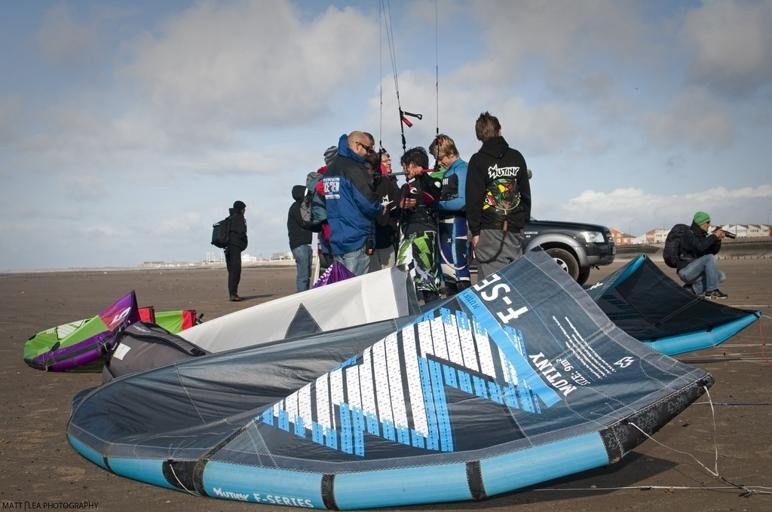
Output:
[467,220,616,286]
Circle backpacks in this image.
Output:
[299,170,330,225]
[663,223,692,267]
[211,217,232,248]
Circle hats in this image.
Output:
[694,212,711,225]
[232,200,245,210]
[401,146,429,168]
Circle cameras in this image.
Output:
[714,226,736,239]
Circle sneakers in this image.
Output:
[229,293,244,301]
[682,284,726,299]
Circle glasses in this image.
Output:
[356,142,370,152]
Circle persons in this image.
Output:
[224,201,247,301]
[677,212,728,299]
[288,111,530,303]
